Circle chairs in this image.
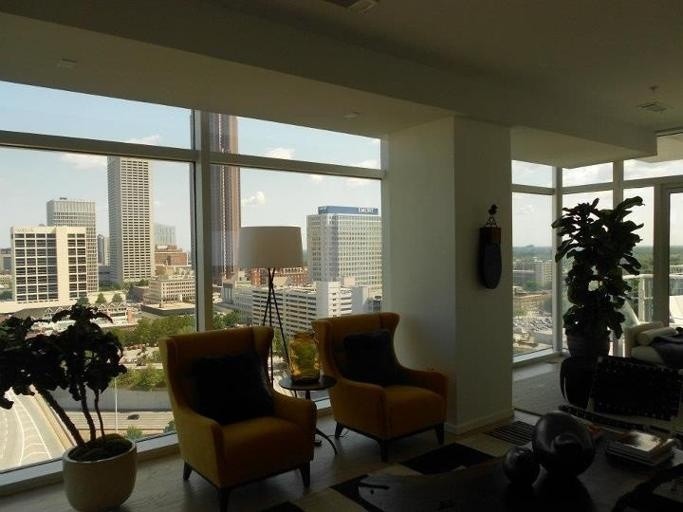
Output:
[158,326,318,511]
[312,312,449,463]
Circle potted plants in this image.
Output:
[548,194,646,358]
[0,300,137,512]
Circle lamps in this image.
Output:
[235,225,322,449]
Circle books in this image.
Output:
[606,430,675,467]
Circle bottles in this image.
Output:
[286,328,320,384]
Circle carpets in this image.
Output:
[263,419,536,512]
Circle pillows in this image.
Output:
[637,327,677,346]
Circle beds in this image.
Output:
[625,319,683,377]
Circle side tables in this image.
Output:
[278,374,339,456]
